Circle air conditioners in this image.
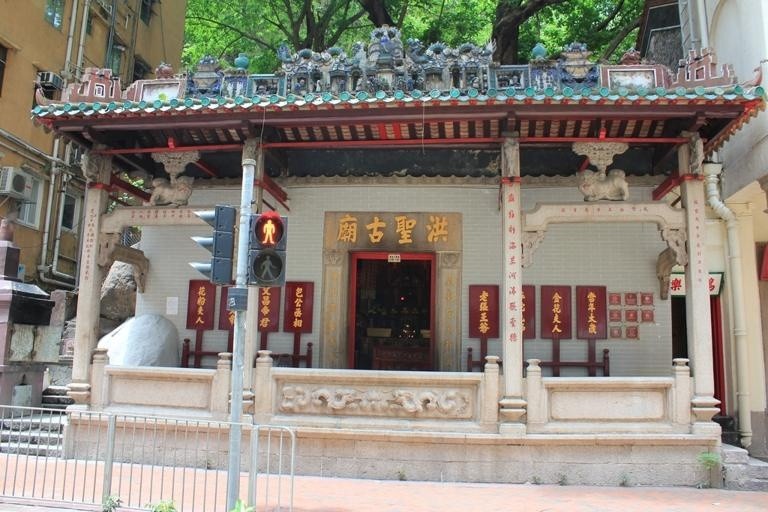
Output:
[40,71,63,89]
[0,166,34,201]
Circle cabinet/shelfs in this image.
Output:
[372,343,428,371]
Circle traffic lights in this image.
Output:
[190,206,237,288]
[244,211,289,287]
